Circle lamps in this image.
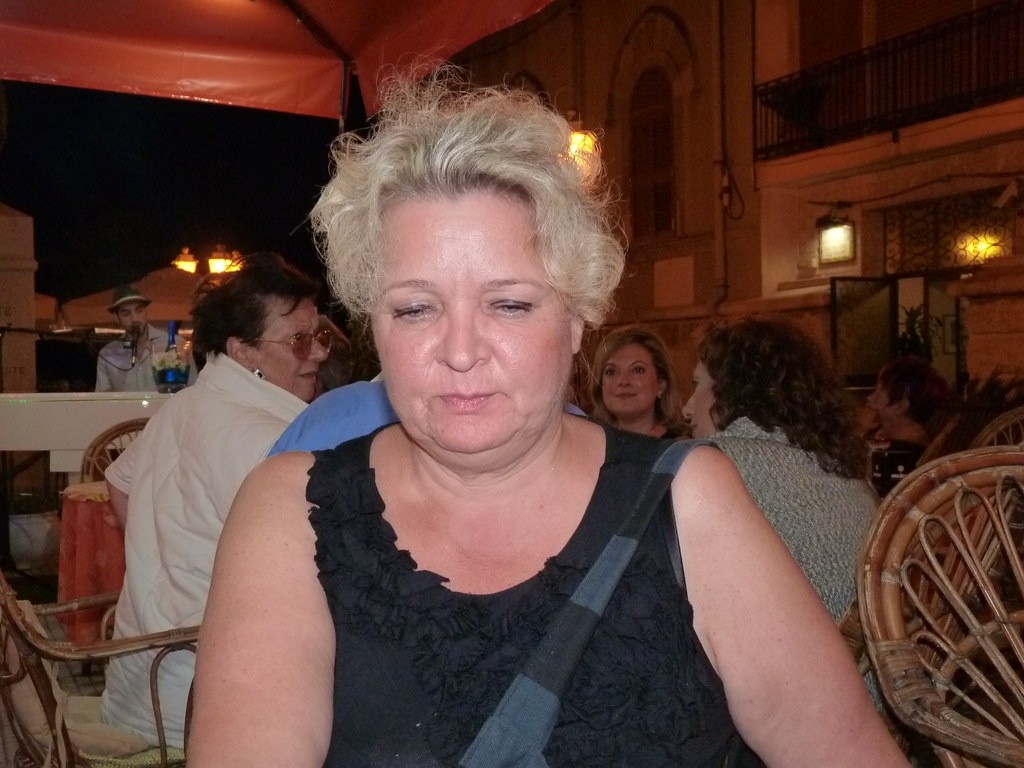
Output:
[171,244,249,274]
[817,215,856,265]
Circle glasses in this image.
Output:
[254,330,331,360]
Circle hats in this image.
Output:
[107,284,151,312]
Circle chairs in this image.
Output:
[82,418,150,481]
[0,570,201,768]
[834,394,1024,768]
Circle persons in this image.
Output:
[589,323,691,440]
[95,281,198,393]
[683,322,903,759]
[865,349,951,502]
[102,251,333,752]
[190,63,913,768]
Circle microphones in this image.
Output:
[131,327,140,367]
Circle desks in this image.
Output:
[58,482,126,645]
